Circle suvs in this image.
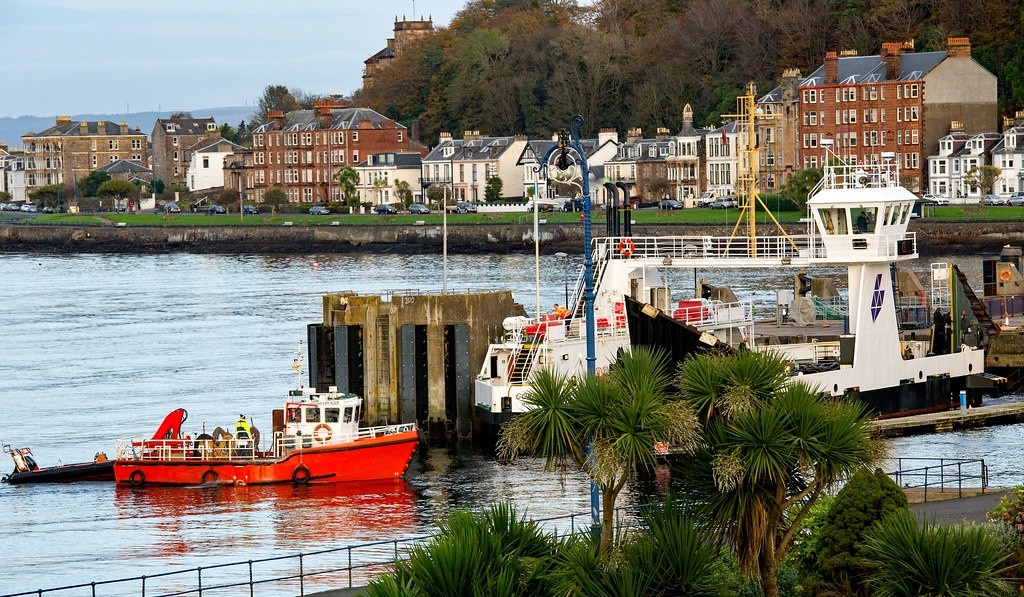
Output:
[115,201,126,212]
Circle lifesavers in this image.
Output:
[619,239,634,256]
[1000,270,1012,282]
[129,469,145,486]
[313,423,333,442]
[202,469,219,484]
[292,467,310,484]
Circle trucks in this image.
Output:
[698,190,738,207]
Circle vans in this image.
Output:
[1007,191,1024,206]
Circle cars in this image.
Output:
[408,203,430,214]
[709,197,738,209]
[209,205,226,214]
[375,204,398,215]
[979,194,1004,206]
[657,199,683,210]
[164,204,180,214]
[244,204,259,214]
[309,206,330,215]
[527,196,643,212]
[0,202,37,213]
[922,193,949,207]
[456,202,477,214]
[42,206,53,214]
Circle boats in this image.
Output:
[0,442,132,485]
[112,338,419,489]
[473,78,1001,438]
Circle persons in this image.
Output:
[960,309,971,331]
[236,414,252,460]
[739,336,751,354]
[554,303,572,337]
[778,303,789,324]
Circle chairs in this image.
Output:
[525,300,710,342]
